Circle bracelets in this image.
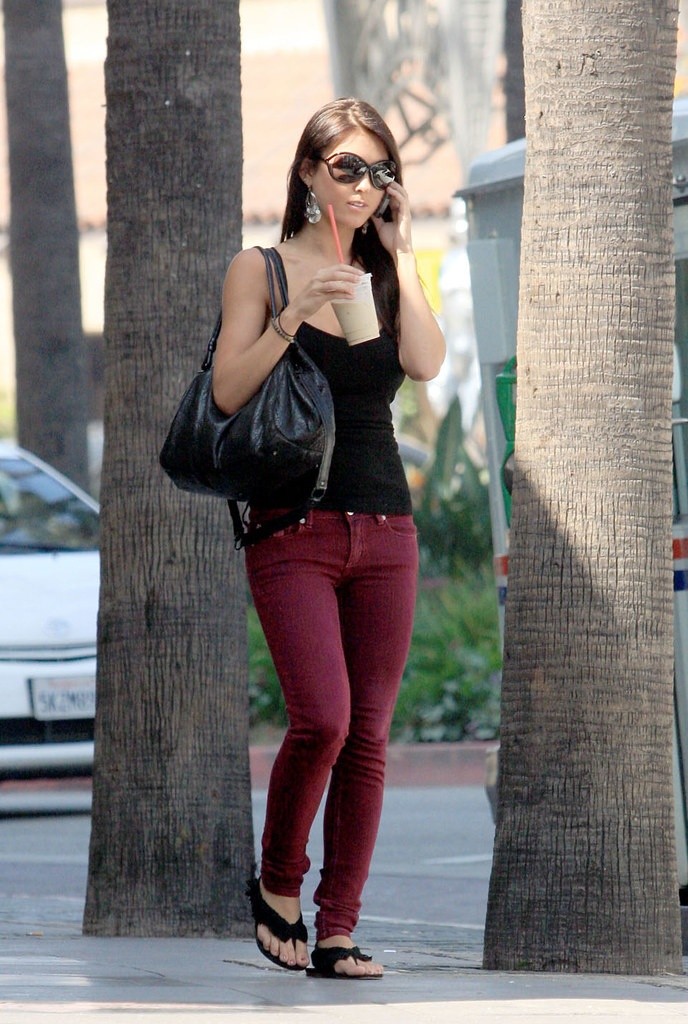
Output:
[271,314,295,343]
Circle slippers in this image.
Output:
[251,873,310,971]
[304,940,384,981]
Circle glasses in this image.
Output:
[317,152,398,190]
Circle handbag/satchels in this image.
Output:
[157,246,336,551]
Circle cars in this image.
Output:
[0,443,100,814]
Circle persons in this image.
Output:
[212,97,446,979]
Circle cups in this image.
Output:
[330,273,381,347]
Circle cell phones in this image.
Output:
[374,191,392,218]
